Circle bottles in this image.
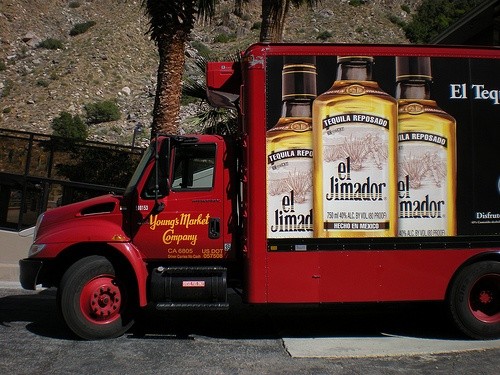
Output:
[313,55,398,238]
[394,55,457,236]
[267,55,318,240]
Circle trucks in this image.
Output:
[17,43,499,342]
